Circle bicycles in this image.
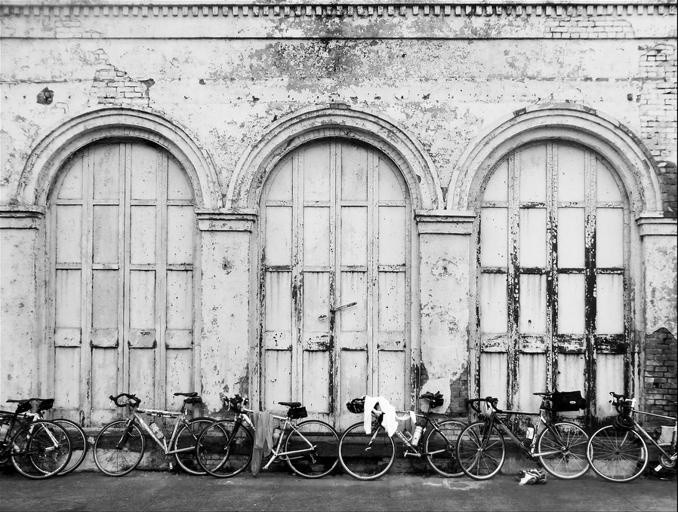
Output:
[0,398,86,476]
[456,391,594,481]
[0,399,71,480]
[197,394,340,479]
[338,392,481,481]
[585,391,677,482]
[92,392,230,477]
